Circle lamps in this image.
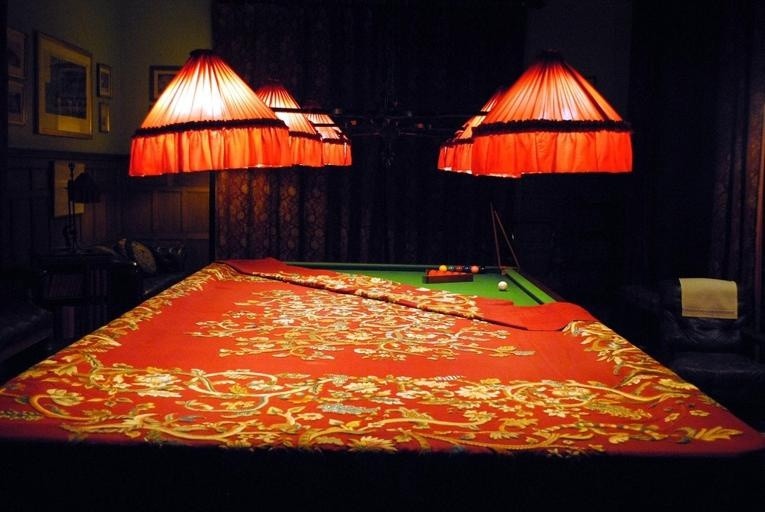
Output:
[250,78,324,173]
[434,53,634,177]
[126,49,292,176]
[300,97,354,171]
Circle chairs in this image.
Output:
[647,272,762,400]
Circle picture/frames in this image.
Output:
[6,26,114,138]
[149,62,183,102]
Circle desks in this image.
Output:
[0,261,764,477]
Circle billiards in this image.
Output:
[439,264,489,274]
[498,281,508,290]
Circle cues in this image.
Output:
[490,201,502,267]
[494,209,522,267]
